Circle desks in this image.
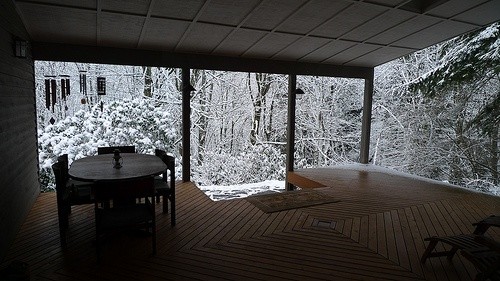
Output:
[67,151,170,245]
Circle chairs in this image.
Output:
[93,192,154,260]
[413,215,499,281]
[50,162,113,250]
[97,144,136,155]
[134,153,176,232]
